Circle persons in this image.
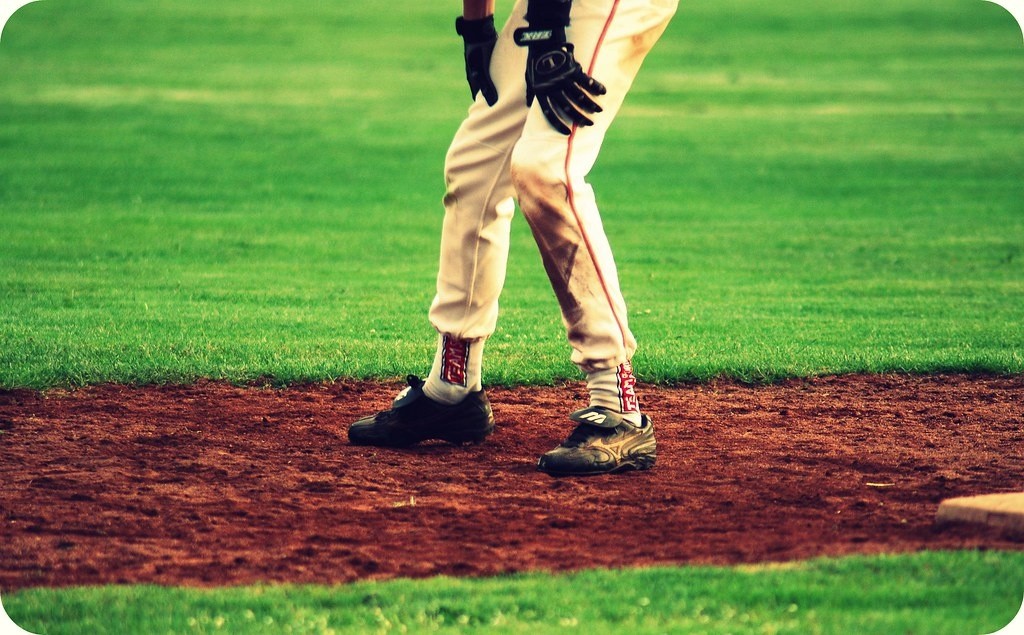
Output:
[348,0,681,478]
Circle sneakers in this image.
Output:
[347,373,496,447]
[535,406,657,477]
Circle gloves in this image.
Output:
[455,14,498,107]
[513,1,606,136]
